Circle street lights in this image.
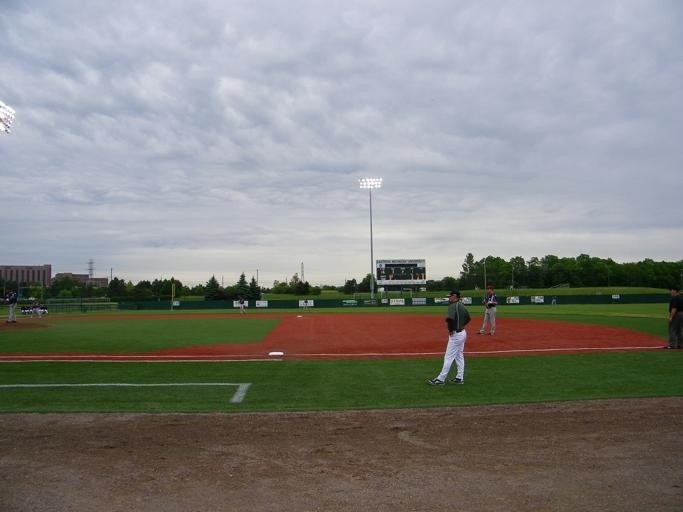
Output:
[358,178,382,299]
[0,100,15,137]
[482,261,486,298]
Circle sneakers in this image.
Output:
[477,330,496,336]
[448,378,466,384]
[664,344,683,349]
[426,378,446,386]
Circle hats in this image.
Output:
[486,284,496,289]
[449,289,461,298]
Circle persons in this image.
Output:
[6,290,17,323]
[427,291,471,385]
[21,301,48,318]
[664,287,683,349]
[552,296,557,304]
[304,299,308,308]
[239,297,245,313]
[477,286,498,336]
